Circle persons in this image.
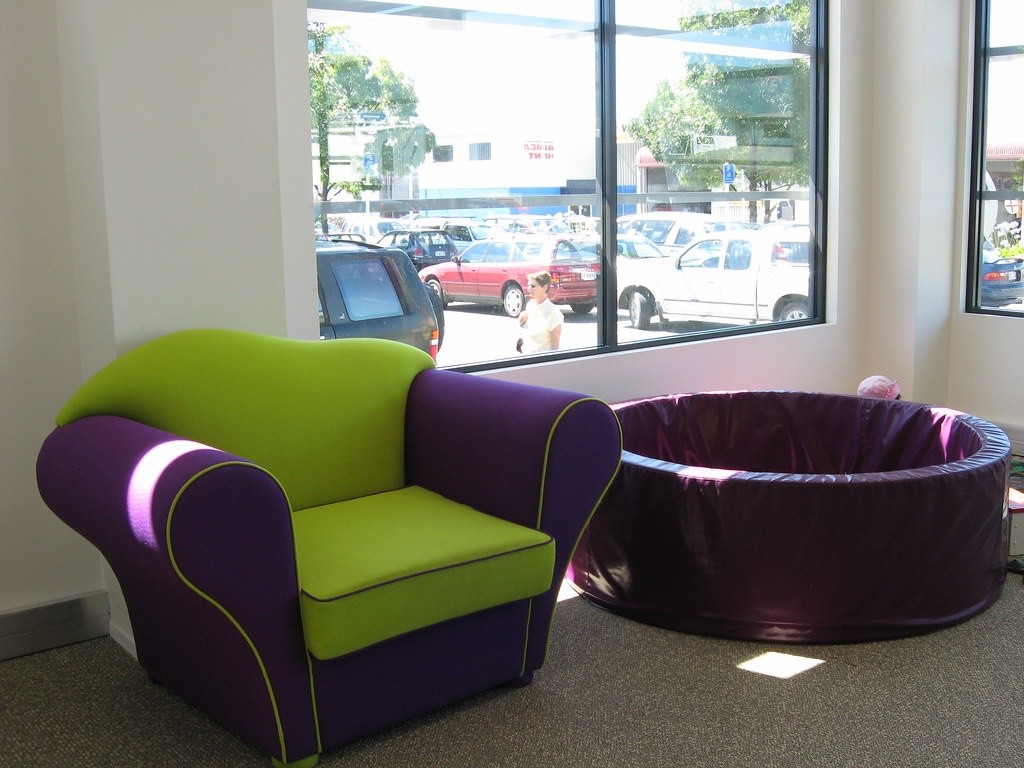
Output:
[519,271,563,354]
[857,375,901,400]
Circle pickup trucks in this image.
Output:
[617,229,810,329]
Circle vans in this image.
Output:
[615,211,718,255]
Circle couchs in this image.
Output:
[34,325,625,767]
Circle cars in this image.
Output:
[418,233,602,319]
[338,209,602,273]
[977,237,1024,307]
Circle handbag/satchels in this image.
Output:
[516,338,523,353]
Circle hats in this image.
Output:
[857,375,900,400]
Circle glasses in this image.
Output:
[527,281,536,288]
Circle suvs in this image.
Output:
[315,233,441,359]
